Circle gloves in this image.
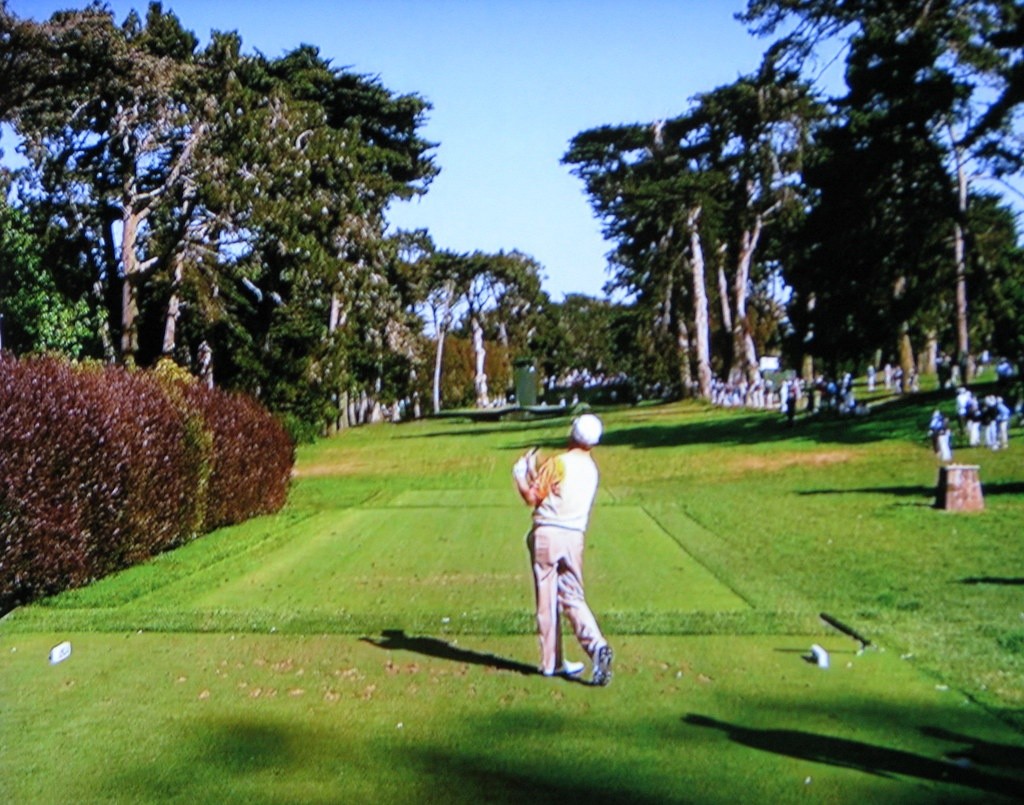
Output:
[512,456,527,480]
[524,447,538,473]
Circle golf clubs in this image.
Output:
[525,402,591,460]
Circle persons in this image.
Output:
[513,411,612,684]
[695,340,1024,469]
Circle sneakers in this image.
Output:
[587,640,614,687]
[542,658,585,677]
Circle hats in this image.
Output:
[571,414,603,445]
